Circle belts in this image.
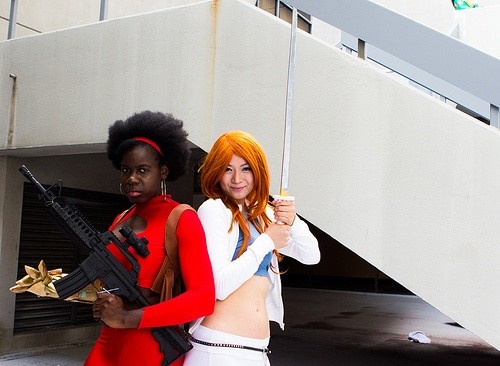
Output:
[188,334,271,354]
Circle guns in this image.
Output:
[19,164,193,366]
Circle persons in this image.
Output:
[183,130,322,366]
[84,109,217,366]
